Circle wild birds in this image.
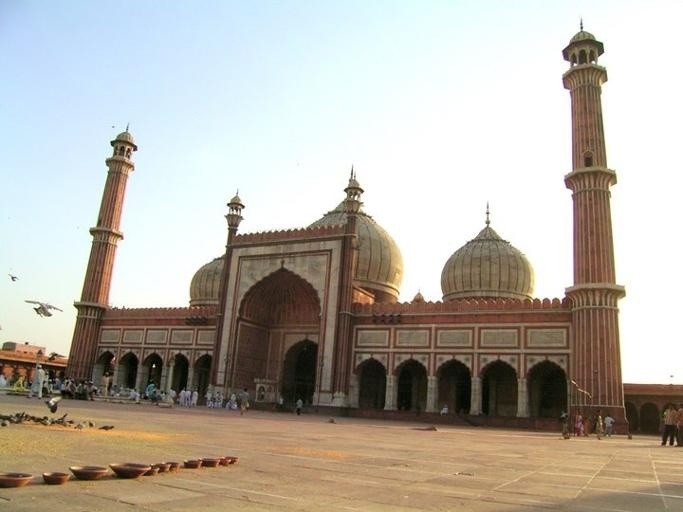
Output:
[44,396,61,413]
[7,273,18,282]
[571,380,591,400]
[24,299,62,317]
[47,352,58,362]
[0,412,96,432]
[99,425,114,431]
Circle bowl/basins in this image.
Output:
[110,455,238,478]
[68,466,108,479]
[42,471,69,484]
[0,471,32,486]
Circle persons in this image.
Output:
[277,393,284,410]
[440,402,448,415]
[660,403,680,446]
[675,404,683,446]
[559,409,616,439]
[0,363,251,416]
[295,396,302,414]
